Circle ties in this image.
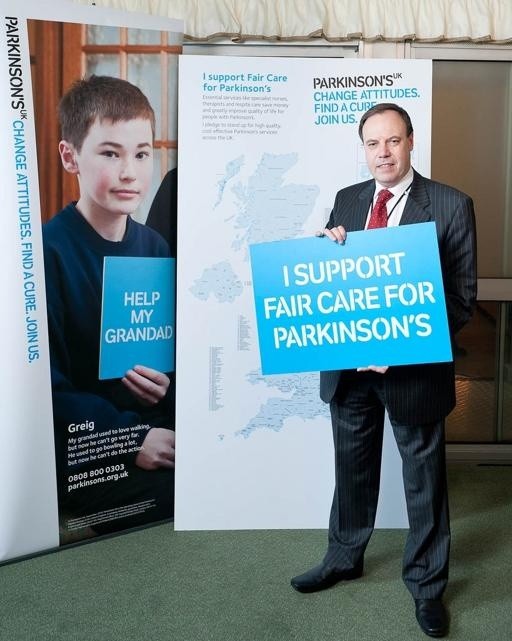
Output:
[366,190,393,231]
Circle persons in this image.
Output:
[289,103,478,638]
[38,73,176,543]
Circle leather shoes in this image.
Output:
[290,559,363,592]
[414,598,450,637]
